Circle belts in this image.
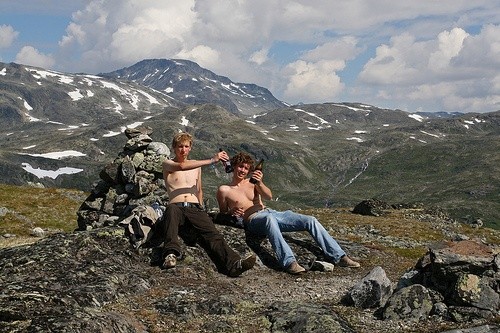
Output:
[175,202,198,208]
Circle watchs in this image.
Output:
[217,151,360,274]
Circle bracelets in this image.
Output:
[210,158,214,163]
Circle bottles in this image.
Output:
[249,158,264,184]
[219,148,233,173]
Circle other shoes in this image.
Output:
[229,255,256,277]
[339,255,360,267]
[163,253,177,268]
[287,261,305,273]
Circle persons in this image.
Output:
[161,131,256,278]
